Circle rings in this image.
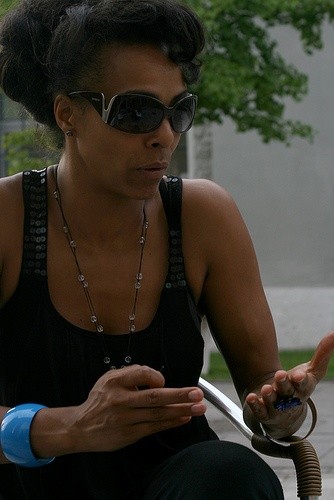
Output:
[273,383,302,410]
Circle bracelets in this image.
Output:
[260,397,317,446]
[1,403,58,467]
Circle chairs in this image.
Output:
[197,377,322,500]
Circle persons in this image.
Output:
[0,0,332,500]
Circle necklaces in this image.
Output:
[53,165,149,370]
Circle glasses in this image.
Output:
[68,91,198,134]
